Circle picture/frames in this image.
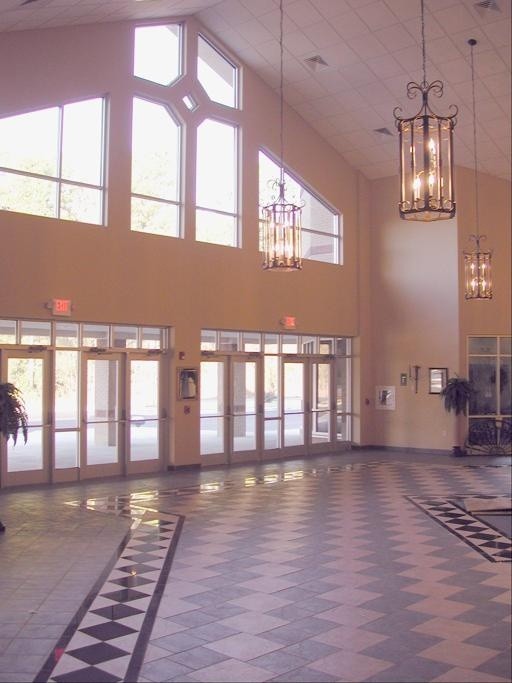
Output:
[375,386,395,411]
[177,368,199,400]
[428,367,448,394]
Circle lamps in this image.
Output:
[261,0,302,273]
[394,0,458,221]
[462,40,492,299]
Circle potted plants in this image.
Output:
[440,377,480,457]
